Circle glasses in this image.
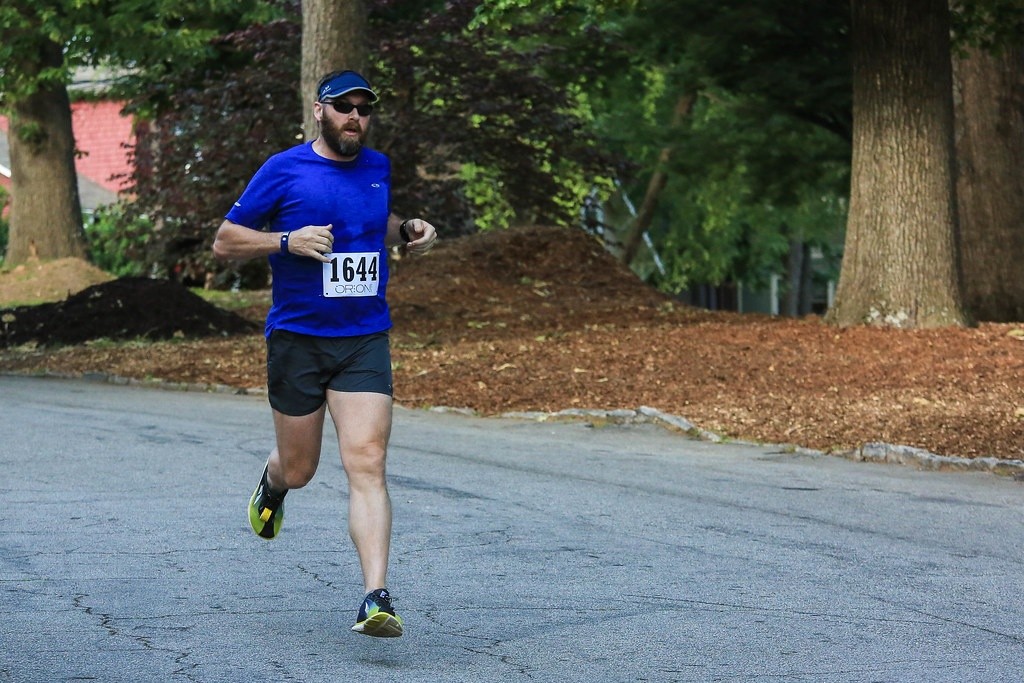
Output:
[321,101,373,117]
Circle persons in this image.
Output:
[212,71,437,637]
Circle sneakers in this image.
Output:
[351,589,404,637]
[248,464,288,540]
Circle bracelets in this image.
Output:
[280,230,294,255]
[400,220,412,243]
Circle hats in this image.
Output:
[317,70,377,102]
